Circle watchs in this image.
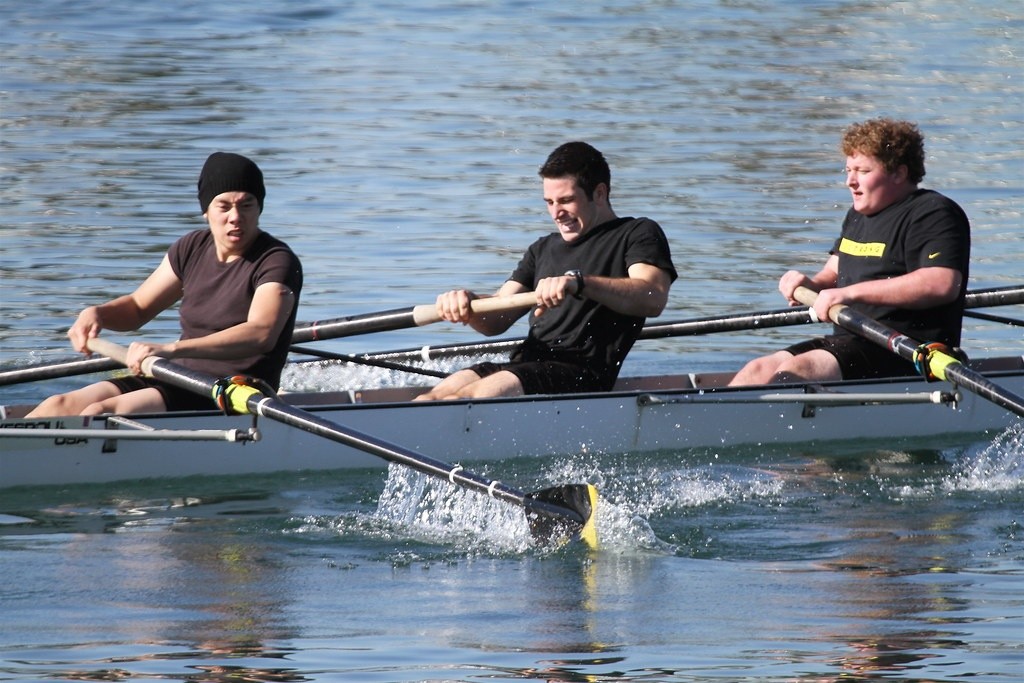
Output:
[564,269,586,300]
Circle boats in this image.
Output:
[0,355,1023,489]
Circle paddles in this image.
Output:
[794,284,1024,417]
[292,283,1024,369]
[0,291,544,387]
[85,335,600,552]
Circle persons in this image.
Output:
[726,119,970,387]
[23,151,302,419]
[410,141,678,402]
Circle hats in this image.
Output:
[197,152,266,214]
